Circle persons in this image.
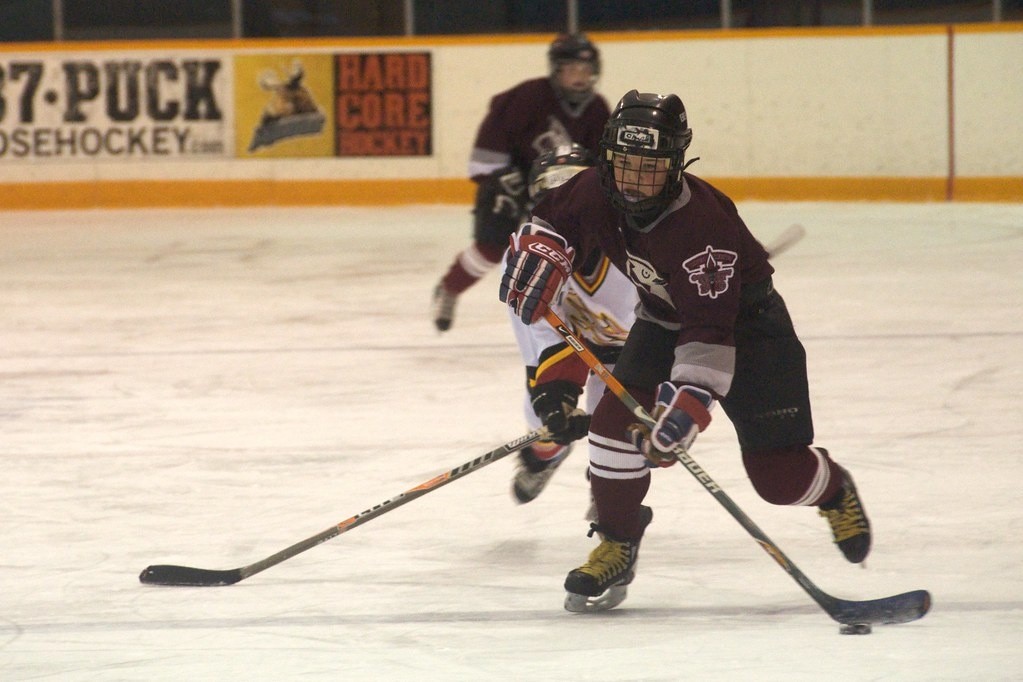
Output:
[430,32,612,332]
[502,143,641,542]
[498,89,873,614]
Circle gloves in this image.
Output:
[499,222,576,325]
[531,379,592,443]
[625,381,713,468]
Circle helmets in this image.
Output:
[597,88,692,216]
[528,141,594,203]
[550,33,600,118]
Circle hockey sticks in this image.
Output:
[135,406,586,589]
[539,304,935,629]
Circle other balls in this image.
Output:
[838,624,872,636]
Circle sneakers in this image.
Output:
[819,467,871,568]
[513,447,571,501]
[564,522,640,613]
[434,284,454,332]
